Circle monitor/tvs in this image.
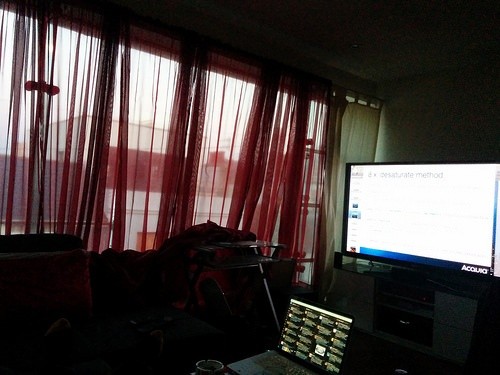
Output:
[341,161,500,285]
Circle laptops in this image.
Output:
[226,294,355,375]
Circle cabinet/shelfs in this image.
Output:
[194,240,286,334]
[334,263,489,364]
